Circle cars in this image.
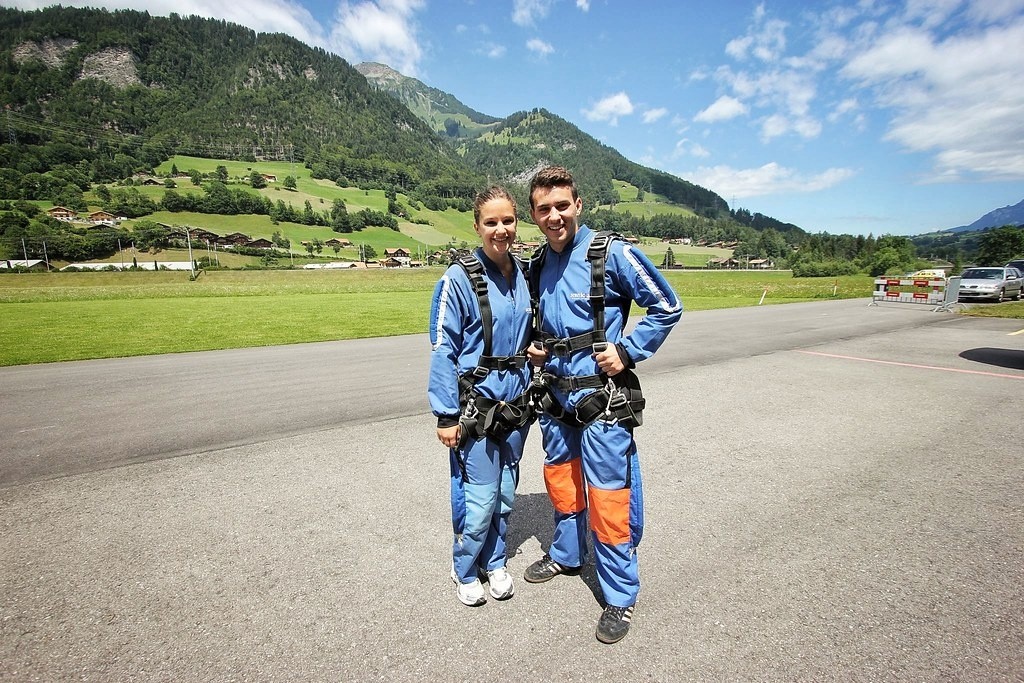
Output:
[1004,259,1024,276]
[953,267,1024,303]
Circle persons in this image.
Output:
[427,185,533,606]
[523,166,683,643]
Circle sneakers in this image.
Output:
[480,565,514,599]
[524,552,582,583]
[596,602,635,643]
[450,560,487,607]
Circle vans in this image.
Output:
[912,270,945,277]
